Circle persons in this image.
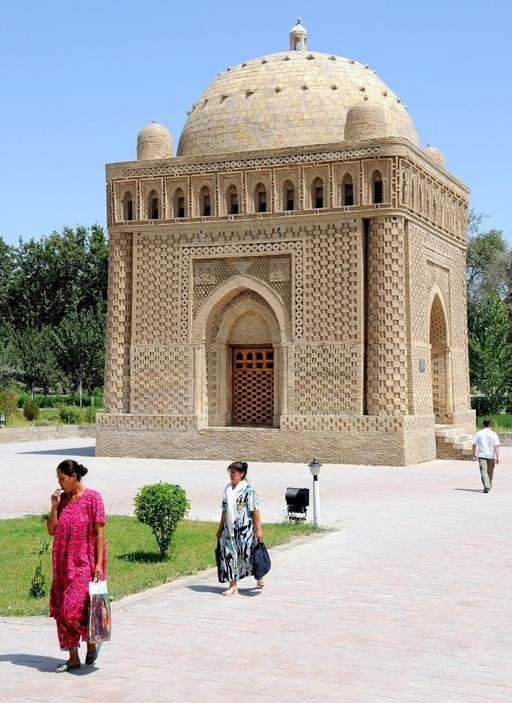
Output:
[47,459,106,673]
[216,462,266,596]
[471,418,500,493]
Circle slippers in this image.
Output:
[85,643,103,665]
[55,660,81,673]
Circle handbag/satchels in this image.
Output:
[250,539,271,581]
[215,537,229,583]
[87,578,112,644]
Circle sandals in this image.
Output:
[257,578,265,589]
[221,586,239,596]
